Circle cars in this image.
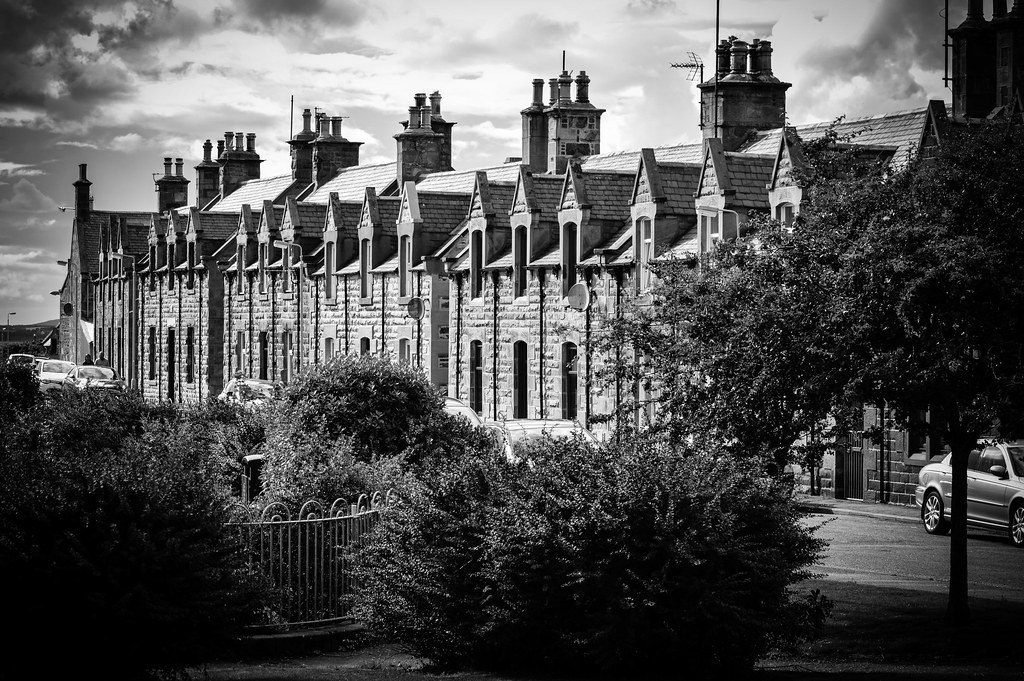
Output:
[916,438,1024,548]
[440,396,481,430]
[61,365,129,396]
[219,376,292,409]
[32,360,77,395]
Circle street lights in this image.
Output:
[7,312,17,353]
[112,251,136,390]
[57,260,77,365]
[273,240,303,374]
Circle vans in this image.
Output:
[7,353,35,368]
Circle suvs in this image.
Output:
[476,418,603,463]
